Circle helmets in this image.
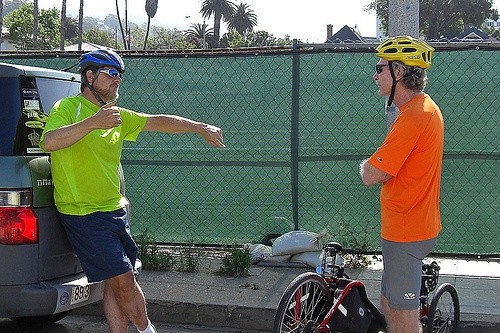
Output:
[78,49,125,73]
[376,35,435,68]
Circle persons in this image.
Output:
[361,36,444,333]
[39,49,226,333]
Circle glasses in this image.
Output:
[100,68,122,81]
[375,63,389,73]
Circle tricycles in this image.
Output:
[271,241,460,333]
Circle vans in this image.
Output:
[0,62,143,326]
